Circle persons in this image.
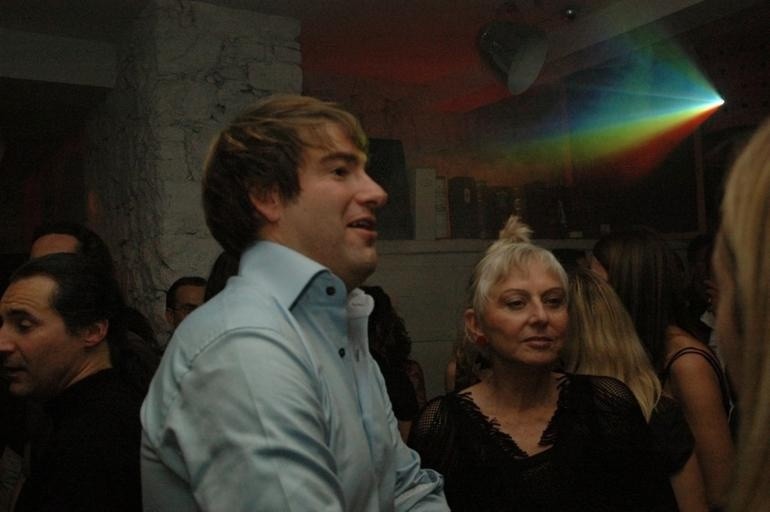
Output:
[138,94,451,512]
[159,275,208,330]
[23,222,161,348]
[206,248,238,300]
[415,217,740,512]
[1,251,150,508]
[359,283,427,445]
[711,121,770,512]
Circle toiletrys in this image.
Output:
[413,167,599,241]
